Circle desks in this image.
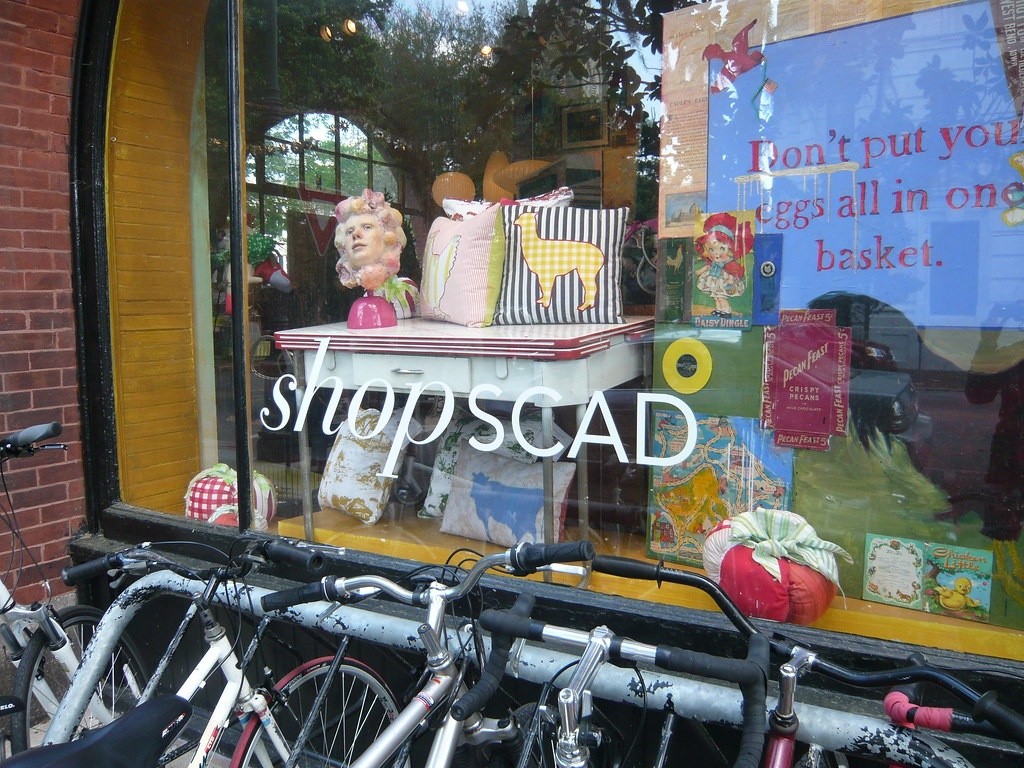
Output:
[274,314,654,585]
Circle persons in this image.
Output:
[963,300,1024,486]
[333,188,408,291]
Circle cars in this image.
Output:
[280,395,873,550]
[775,322,898,372]
[478,324,935,473]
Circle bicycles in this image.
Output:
[0,423,1024,768]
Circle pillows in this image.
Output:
[317,405,421,524]
[441,441,579,549]
[415,406,559,520]
[419,189,633,327]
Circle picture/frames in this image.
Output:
[560,100,610,153]
